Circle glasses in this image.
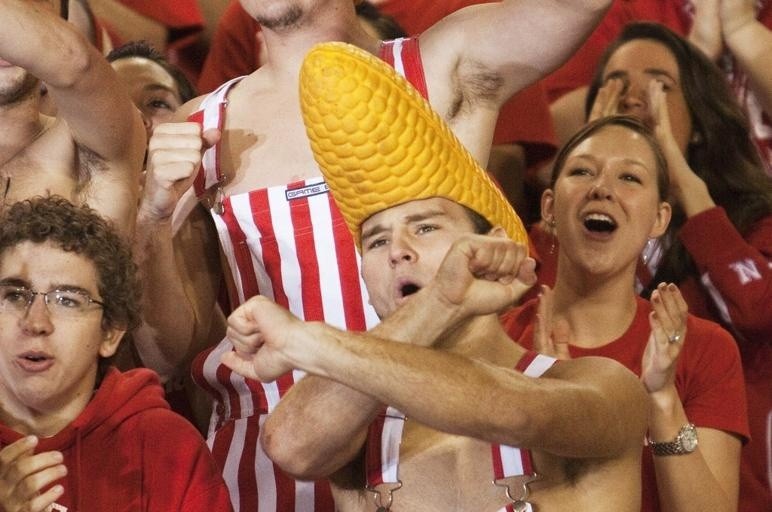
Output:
[1,283,107,321]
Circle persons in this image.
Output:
[1,0,771,511]
